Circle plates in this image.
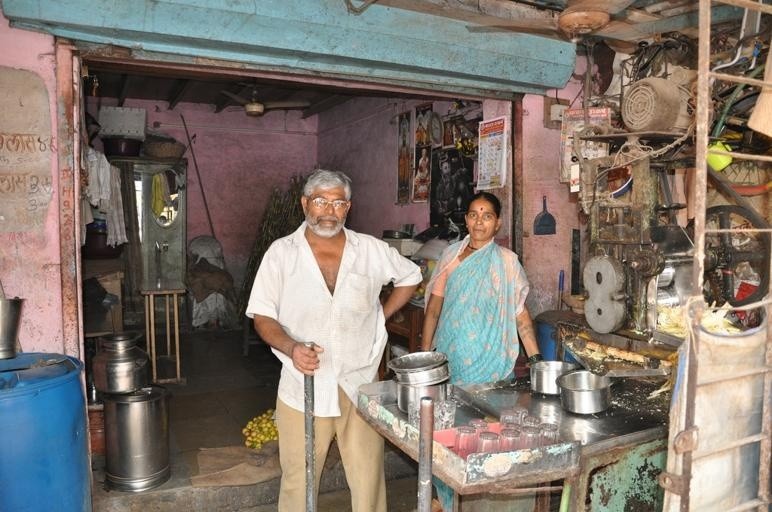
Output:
[386,350,447,374]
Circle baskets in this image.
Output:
[145,142,186,158]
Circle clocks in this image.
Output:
[430,114,445,146]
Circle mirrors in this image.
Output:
[152,169,184,232]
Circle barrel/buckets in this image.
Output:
[0,353,92,512]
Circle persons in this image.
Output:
[242,168,426,512]
[413,190,548,511]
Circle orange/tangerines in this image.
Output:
[242,409,279,449]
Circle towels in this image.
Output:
[152,171,166,219]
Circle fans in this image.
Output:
[219,84,313,118]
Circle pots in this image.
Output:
[530,360,576,395]
[392,374,452,415]
[555,368,613,415]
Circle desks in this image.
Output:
[379,287,428,379]
[140,289,188,386]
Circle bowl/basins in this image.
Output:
[101,137,144,157]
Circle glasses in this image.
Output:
[306,197,350,210]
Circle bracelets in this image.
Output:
[525,353,544,365]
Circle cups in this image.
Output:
[707,140,734,172]
[454,405,559,461]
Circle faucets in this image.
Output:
[155,240,162,255]
